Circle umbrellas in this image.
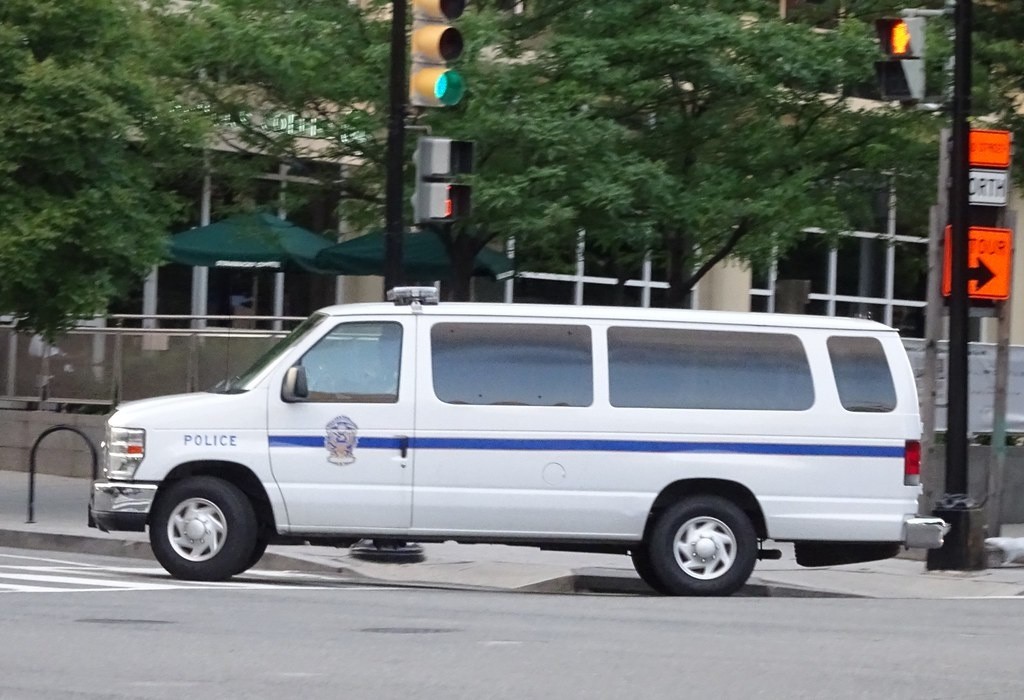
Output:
[314,212,516,286]
[150,208,351,328]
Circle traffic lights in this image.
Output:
[878,59,927,100]
[407,0,470,110]
[873,16,926,57]
[415,135,476,227]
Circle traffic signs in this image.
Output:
[944,224,1011,298]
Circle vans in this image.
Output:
[90,287,947,597]
